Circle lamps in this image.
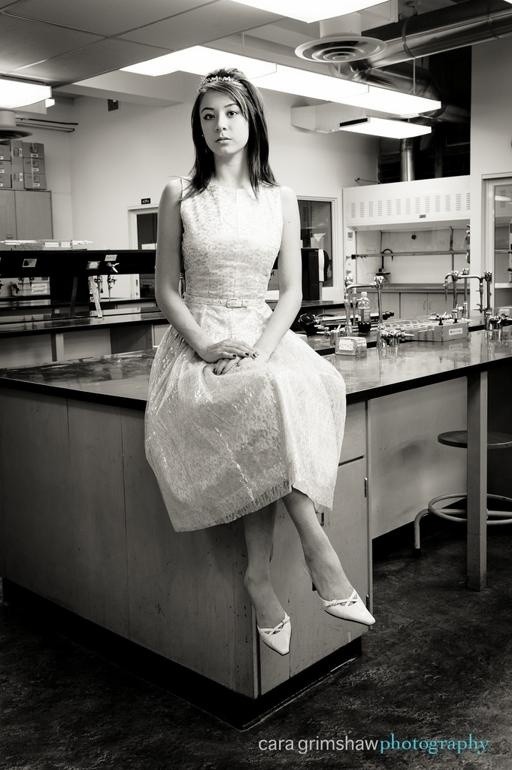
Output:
[1,75,53,111]
[339,118,433,141]
[122,33,443,118]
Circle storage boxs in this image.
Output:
[1,138,48,191]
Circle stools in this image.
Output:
[409,430,511,550]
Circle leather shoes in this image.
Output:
[257,611,291,656]
[311,583,376,626]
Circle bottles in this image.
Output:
[357,291,372,334]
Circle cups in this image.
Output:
[377,333,399,352]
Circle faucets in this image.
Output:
[381,248,394,273]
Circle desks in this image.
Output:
[0,311,512,733]
[0,299,352,370]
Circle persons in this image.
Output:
[142,67,381,658]
